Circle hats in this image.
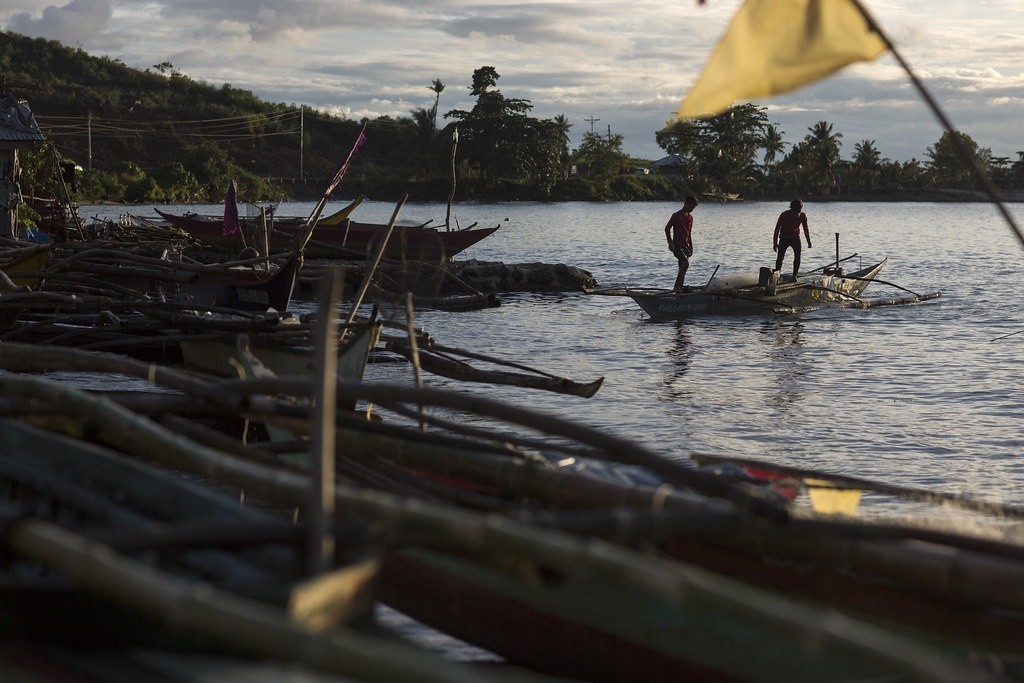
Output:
[788,199,803,209]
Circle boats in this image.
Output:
[623,232,888,322]
[173,295,384,411]
[153,207,501,264]
[0,237,56,331]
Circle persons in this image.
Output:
[665,194,698,293]
[774,198,812,286]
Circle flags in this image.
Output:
[324,129,366,192]
[222,179,241,237]
